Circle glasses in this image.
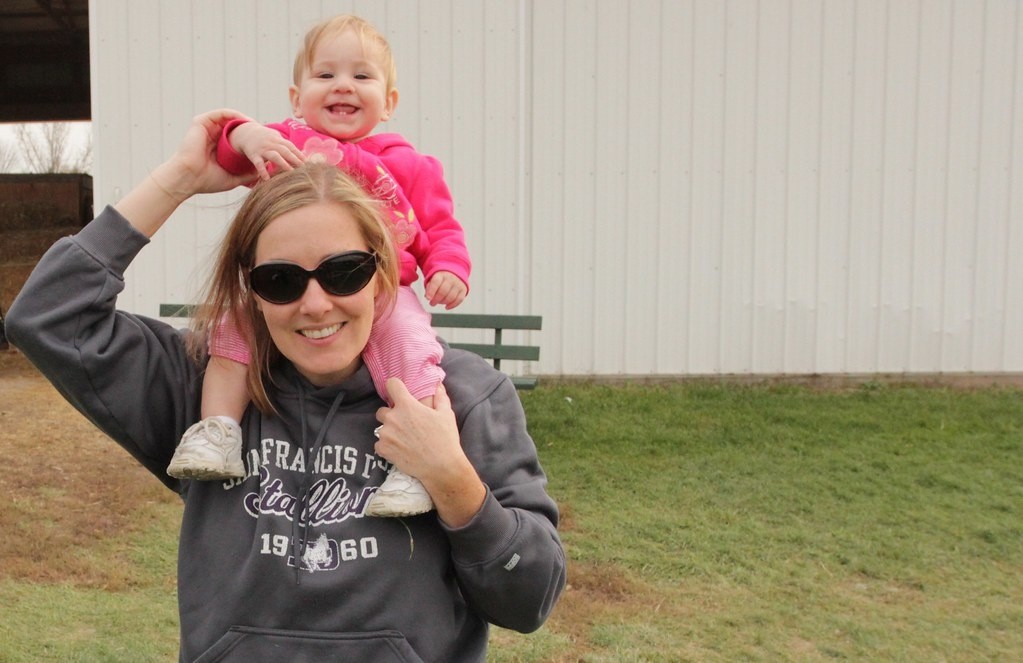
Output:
[249,249,377,304]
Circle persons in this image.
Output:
[165,13,474,518]
[4,108,568,663]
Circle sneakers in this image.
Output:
[166,415,247,480]
[366,463,435,516]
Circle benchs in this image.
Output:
[159,303,543,391]
